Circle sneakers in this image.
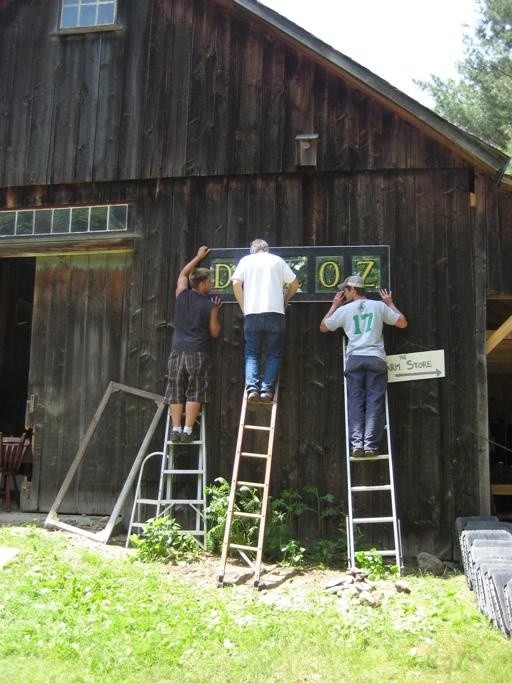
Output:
[248,391,273,402]
[353,448,385,457]
[171,431,196,442]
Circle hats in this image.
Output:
[337,275,364,290]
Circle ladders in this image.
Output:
[342,335,404,579]
[0,204,127,238]
[217,374,279,591]
[157,402,208,552]
[125,452,174,549]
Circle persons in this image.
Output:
[232,237,299,404]
[318,274,410,460]
[162,244,225,445]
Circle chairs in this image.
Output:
[0,431,30,512]
